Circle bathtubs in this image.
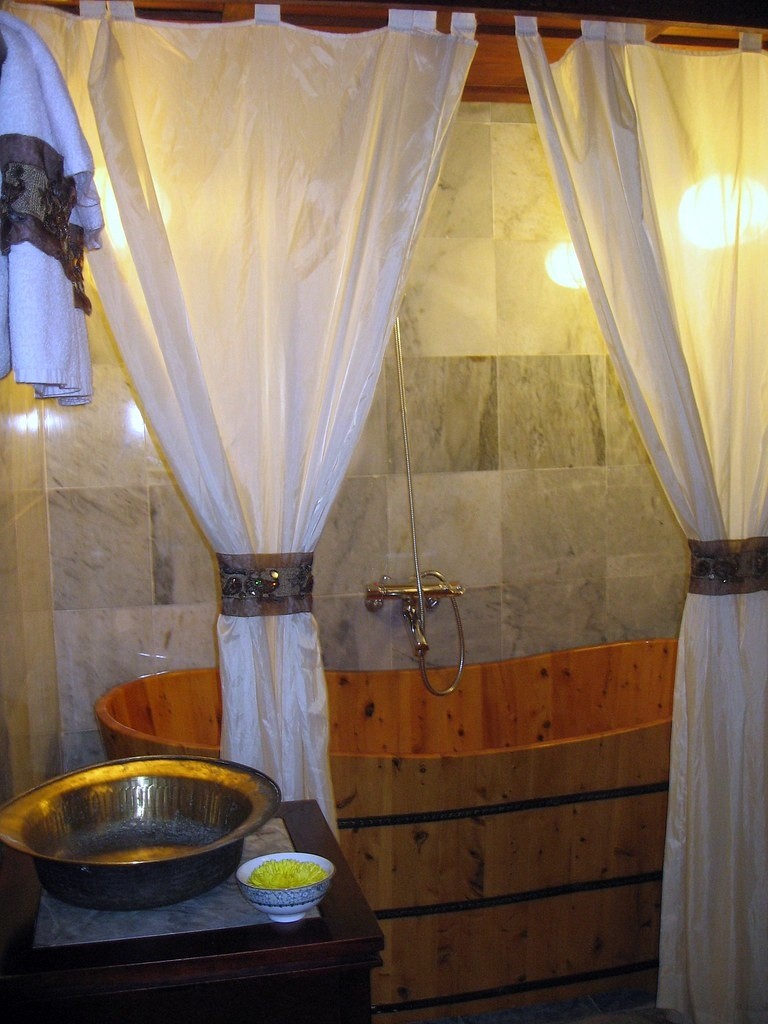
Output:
[94,638,679,1024]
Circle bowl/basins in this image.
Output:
[235,851,336,923]
[0,753,281,910]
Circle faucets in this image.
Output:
[401,597,429,657]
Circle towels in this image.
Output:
[0,10,105,407]
